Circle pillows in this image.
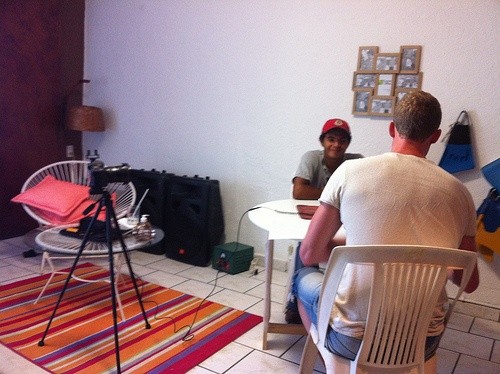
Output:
[9,174,116,225]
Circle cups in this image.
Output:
[126,206,139,224]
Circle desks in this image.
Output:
[249,198,345,351]
[23,223,164,323]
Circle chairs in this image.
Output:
[298,244,478,374]
[22,160,137,274]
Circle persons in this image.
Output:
[290,87,480,374]
[284,116,364,325]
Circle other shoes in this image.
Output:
[284,297,301,324]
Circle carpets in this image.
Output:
[0,261,264,374]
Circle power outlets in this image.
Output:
[66,145,73,157]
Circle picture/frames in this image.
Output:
[351,45,423,117]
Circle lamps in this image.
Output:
[64,105,105,161]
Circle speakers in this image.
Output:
[121,169,227,267]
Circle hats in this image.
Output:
[321,119,349,138]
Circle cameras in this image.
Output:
[87,160,130,189]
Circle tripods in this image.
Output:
[38,189,150,374]
